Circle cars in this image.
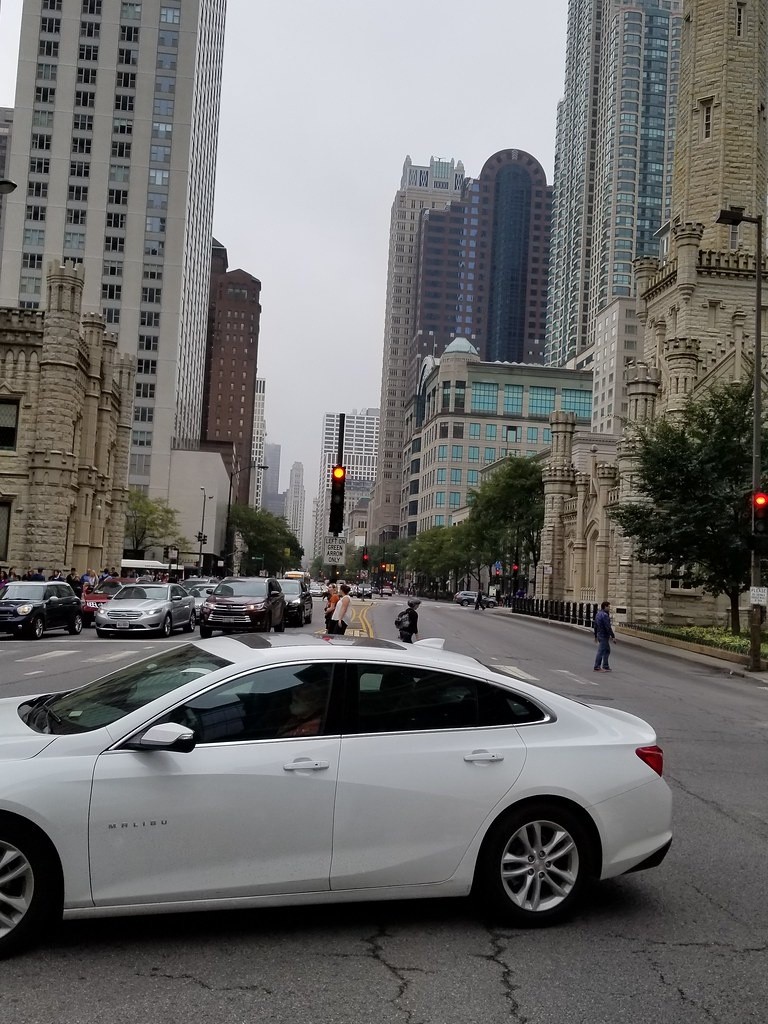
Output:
[276,578,314,629]
[95,584,197,639]
[310,580,393,599]
[0,633,674,959]
[454,591,498,609]
[180,578,219,621]
[0,582,83,641]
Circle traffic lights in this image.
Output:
[198,531,203,542]
[382,563,386,571]
[204,534,207,544]
[513,564,518,576]
[755,493,768,537]
[363,555,369,569]
[163,547,168,558]
[496,570,499,579]
[329,466,346,533]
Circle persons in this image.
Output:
[322,583,339,634]
[330,584,352,635]
[516,587,525,598]
[592,602,617,673]
[0,565,169,587]
[475,588,485,610]
[399,599,421,644]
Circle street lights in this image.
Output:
[198,486,207,576]
[224,465,269,548]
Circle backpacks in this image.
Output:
[394,609,412,630]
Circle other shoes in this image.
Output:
[593,668,605,672]
[603,667,612,672]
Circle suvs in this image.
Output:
[80,576,152,628]
[199,577,287,639]
